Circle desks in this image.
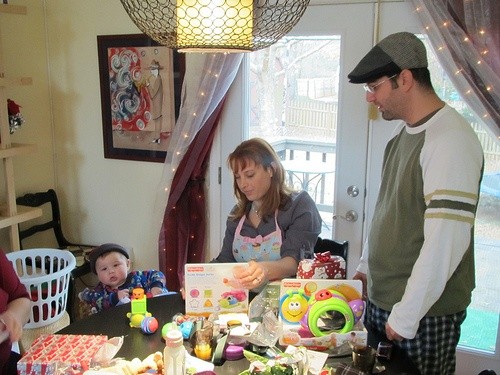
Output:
[55,293,420,375]
[280,159,335,231]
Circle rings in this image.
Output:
[255,278,263,283]
[180,287,184,292]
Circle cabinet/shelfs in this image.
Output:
[0,2,43,275]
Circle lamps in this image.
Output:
[120,0,310,54]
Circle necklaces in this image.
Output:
[251,203,262,215]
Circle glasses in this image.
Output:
[363,74,399,94]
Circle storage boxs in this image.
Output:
[17,331,109,375]
[296,252,346,279]
[277,279,368,356]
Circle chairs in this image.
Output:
[16,188,98,323]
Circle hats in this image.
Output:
[347,32,428,83]
[89,242,129,275]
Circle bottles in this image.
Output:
[163,328,185,375]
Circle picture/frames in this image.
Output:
[97,31,186,164]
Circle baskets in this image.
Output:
[4,248,77,327]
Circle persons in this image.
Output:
[347,31,484,375]
[77,243,168,317]
[0,246,32,375]
[179,137,322,300]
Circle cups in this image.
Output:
[192,319,214,361]
[353,345,377,372]
